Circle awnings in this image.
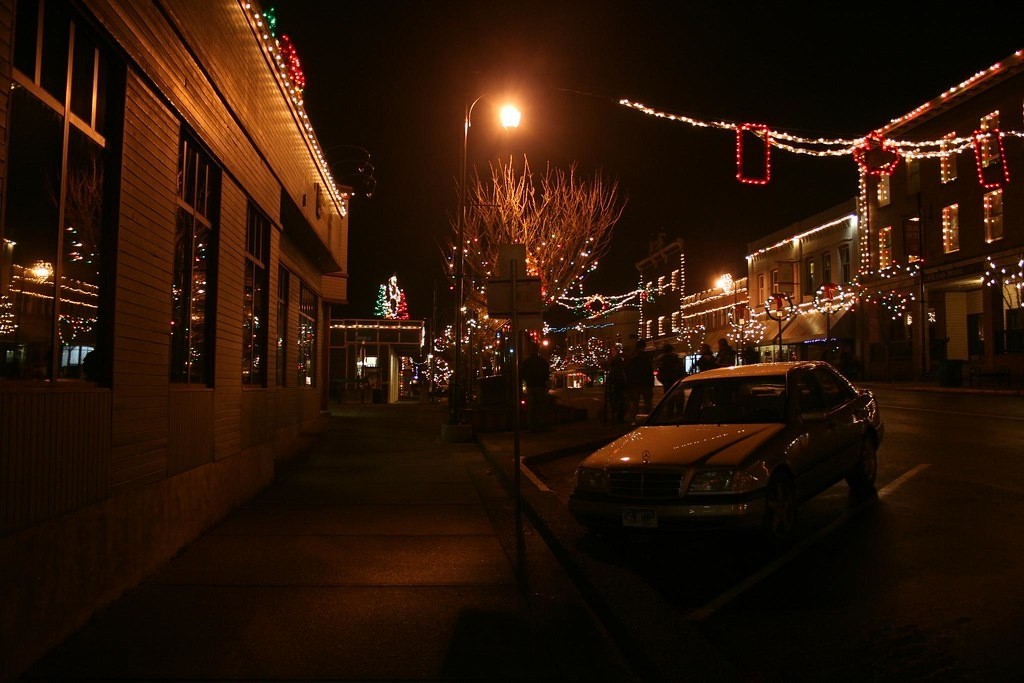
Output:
[695,299,855,352]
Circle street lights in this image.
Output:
[441,86,529,444]
[12,260,54,380]
[716,273,740,366]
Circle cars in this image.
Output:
[571,357,881,571]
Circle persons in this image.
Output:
[609,340,654,424]
[656,344,685,417]
[521,343,550,432]
[713,338,737,368]
[690,344,716,373]
